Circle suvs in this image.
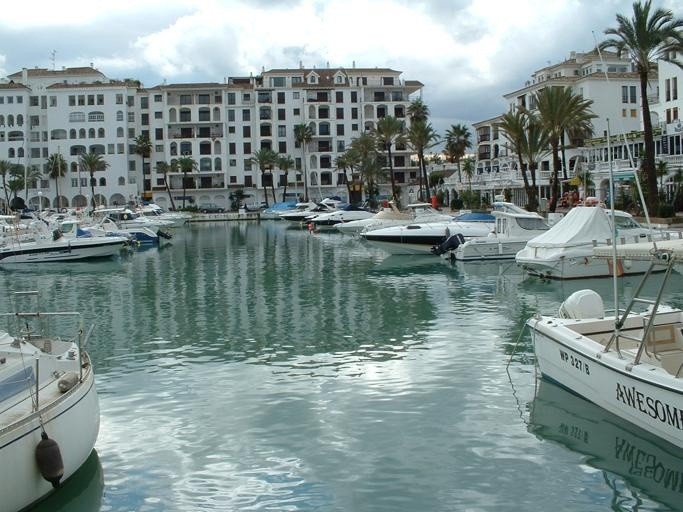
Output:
[199,203,225,213]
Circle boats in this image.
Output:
[526,376,683,512]
[0,291,100,512]
[525,117,683,449]
[0,202,193,263]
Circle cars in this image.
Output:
[262,202,297,213]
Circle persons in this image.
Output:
[504,188,514,202]
[449,193,464,214]
[568,188,579,207]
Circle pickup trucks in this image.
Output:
[240,201,266,212]
